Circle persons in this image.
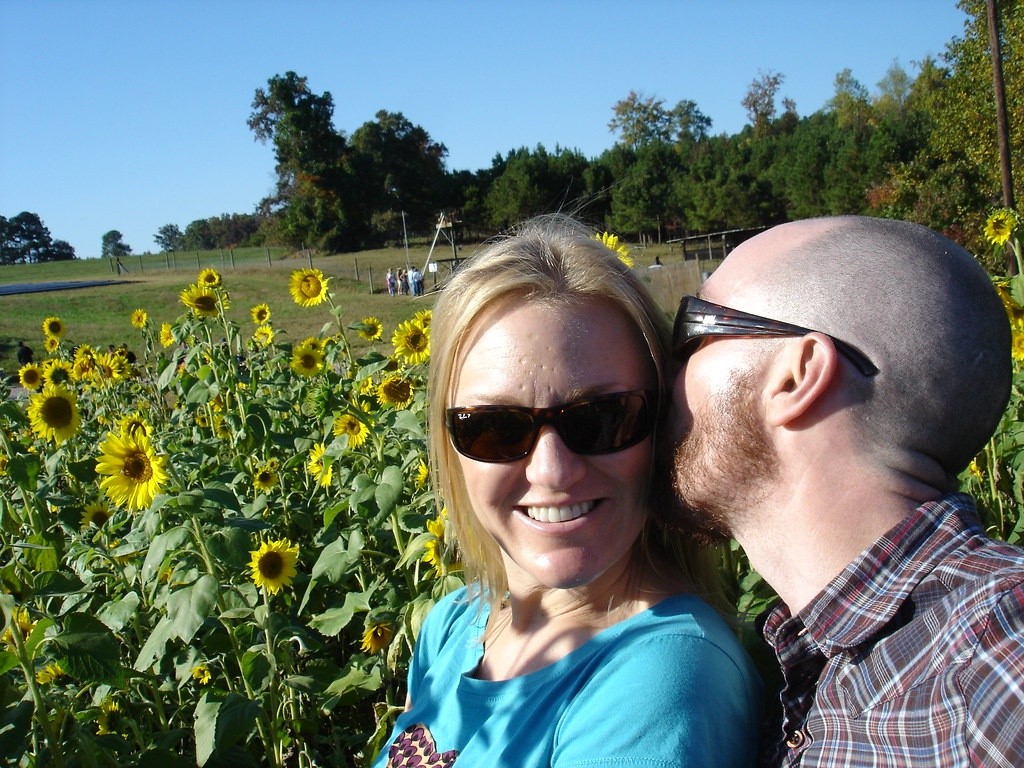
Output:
[386,266,425,297]
[646,214,1023,768]
[372,210,763,768]
[108,343,136,368]
[17,340,33,370]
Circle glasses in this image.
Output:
[445,390,659,464]
[670,295,880,377]
